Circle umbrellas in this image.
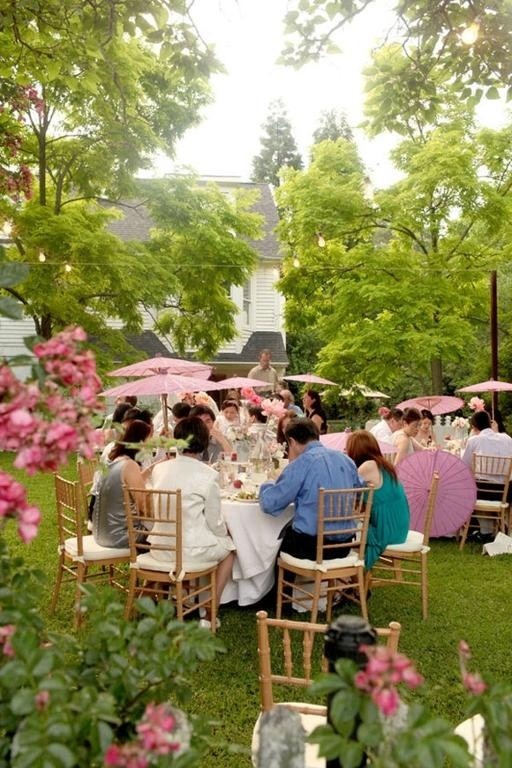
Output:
[105,352,214,378]
[394,450,476,536]
[455,378,512,422]
[283,372,339,414]
[97,372,230,437]
[395,395,464,417]
[319,381,391,399]
[216,373,274,402]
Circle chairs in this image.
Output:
[383,449,395,465]
[76,457,105,571]
[248,609,402,768]
[49,472,139,631]
[458,452,510,554]
[118,485,222,636]
[365,472,444,622]
[276,485,371,628]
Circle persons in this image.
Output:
[248,405,267,424]
[189,404,232,463]
[414,410,433,446]
[461,412,512,542]
[277,409,300,459]
[240,387,265,406]
[91,420,151,550]
[160,403,192,436]
[279,389,304,416]
[346,430,409,600]
[111,404,133,427]
[215,400,245,431]
[102,395,138,429]
[107,408,141,441]
[248,349,278,387]
[146,417,236,631]
[259,418,367,615]
[89,407,155,522]
[387,408,436,467]
[302,391,328,436]
[370,409,403,442]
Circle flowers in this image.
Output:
[239,384,263,406]
[379,405,389,416]
[175,389,209,408]
[254,398,285,458]
[468,396,485,416]
[443,416,467,445]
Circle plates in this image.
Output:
[231,495,263,503]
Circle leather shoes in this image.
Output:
[466,532,495,543]
[198,617,221,630]
